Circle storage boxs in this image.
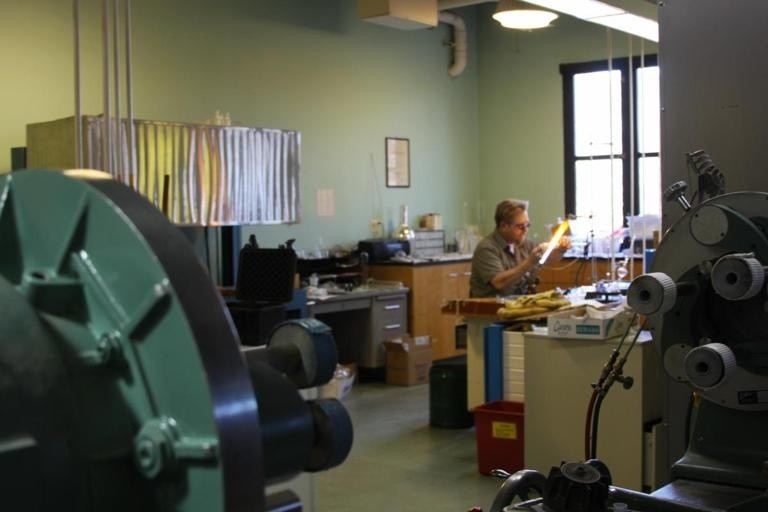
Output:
[382,334,436,385]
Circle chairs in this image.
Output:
[223,247,296,343]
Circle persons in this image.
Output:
[468,198,571,298]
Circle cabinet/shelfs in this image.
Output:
[522,330,696,494]
[444,292,509,413]
[307,282,409,367]
[367,253,474,362]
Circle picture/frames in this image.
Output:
[386,137,410,188]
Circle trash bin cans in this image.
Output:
[471,400,524,476]
[429,356,470,430]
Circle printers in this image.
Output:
[357,238,410,261]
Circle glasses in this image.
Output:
[515,223,531,229]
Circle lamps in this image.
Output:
[492,1,558,30]
[362,0,439,32]
